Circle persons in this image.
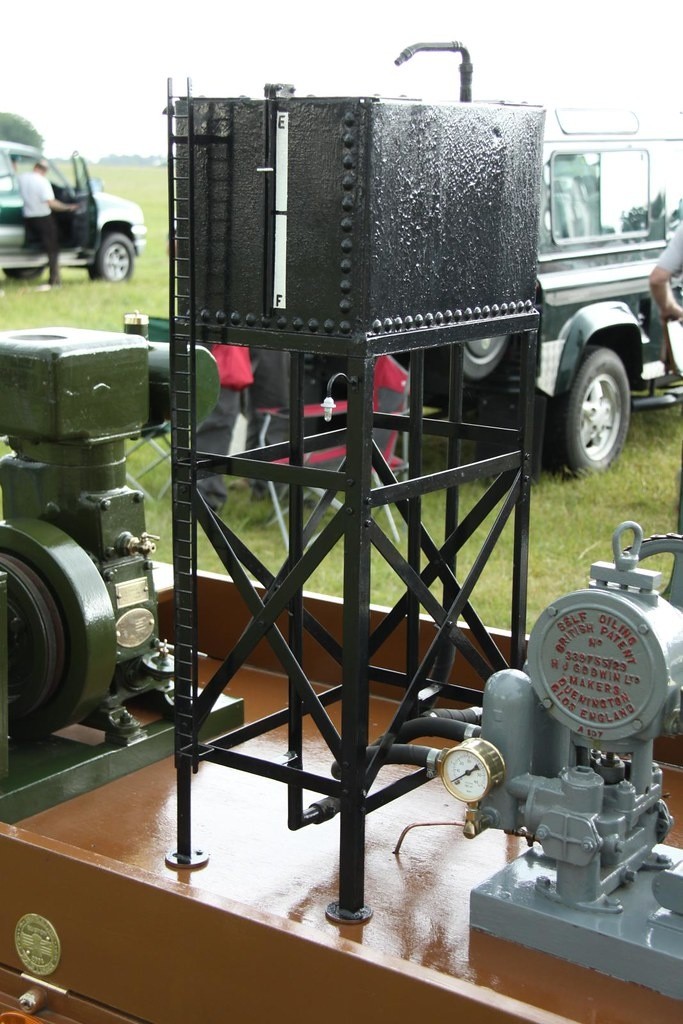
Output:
[195,341,317,513]
[649,222,683,326]
[18,160,80,285]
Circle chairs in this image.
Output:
[255,354,409,554]
[124,345,254,503]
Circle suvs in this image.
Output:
[126,110,683,482]
[1,142,148,286]
